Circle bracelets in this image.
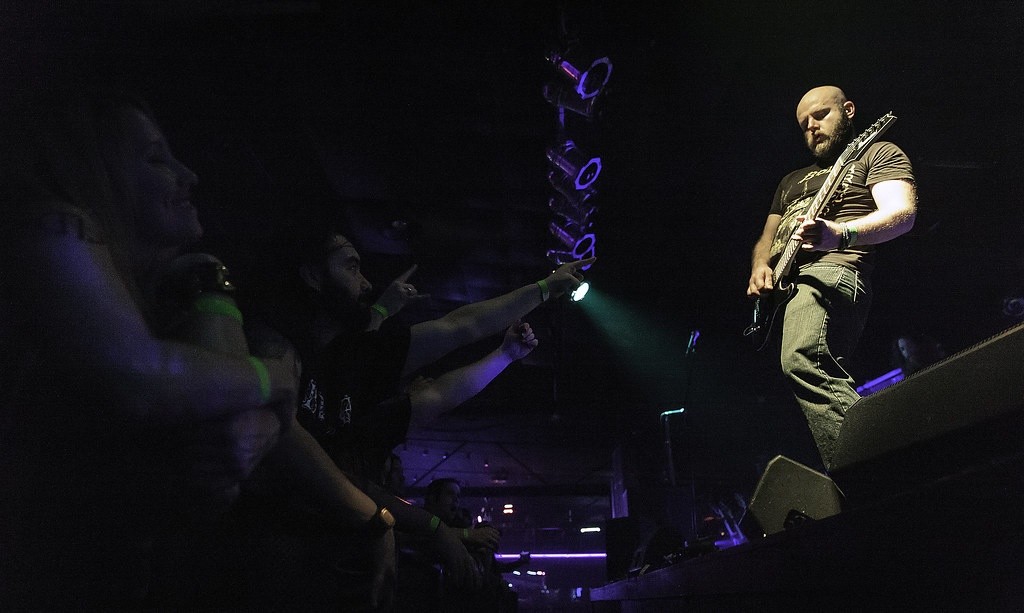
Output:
[537,280,550,301]
[182,262,237,297]
[372,304,389,320]
[245,356,272,402]
[428,514,441,528]
[840,222,850,251]
[190,296,244,324]
[461,528,471,541]
[847,223,858,247]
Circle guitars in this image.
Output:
[748,109,899,354]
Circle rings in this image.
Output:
[407,288,412,294]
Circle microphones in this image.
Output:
[684,329,700,357]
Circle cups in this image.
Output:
[520,551,531,567]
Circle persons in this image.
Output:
[746,85,919,494]
[0,85,598,613]
[893,332,935,371]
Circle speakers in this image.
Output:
[829,325,1023,510]
[738,455,839,536]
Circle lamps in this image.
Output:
[545,36,612,100]
[544,139,601,189]
[547,212,596,258]
[562,279,590,304]
[549,195,598,229]
[547,248,595,271]
[547,167,596,207]
[544,85,602,119]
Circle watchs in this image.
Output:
[360,504,397,541]
